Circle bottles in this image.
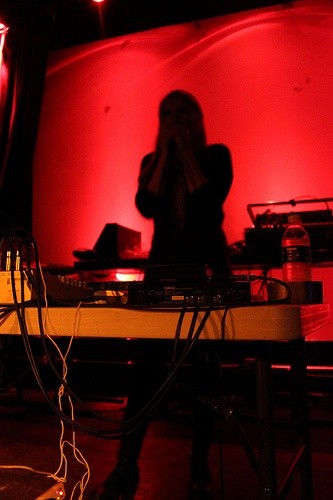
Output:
[282,215,311,303]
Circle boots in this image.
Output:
[189,453,213,500]
[90,449,139,500]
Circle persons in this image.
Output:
[90,89,232,500]
